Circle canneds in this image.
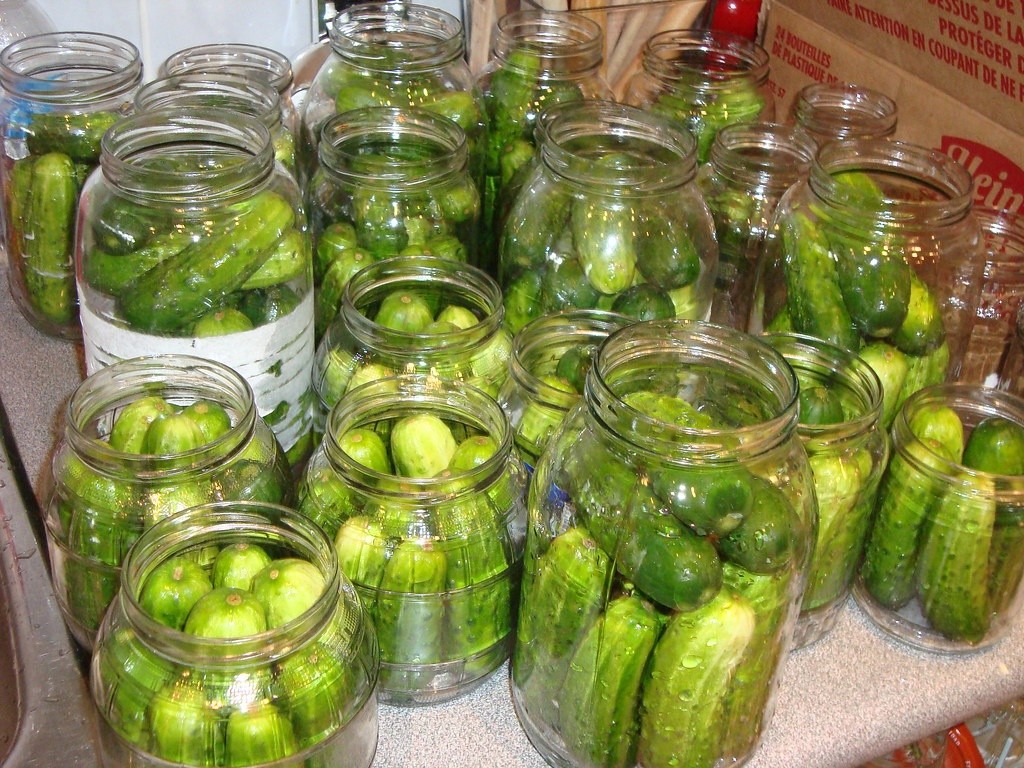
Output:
[0,2,1024,768]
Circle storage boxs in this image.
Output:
[765,0,1024,395]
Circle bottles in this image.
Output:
[0,0,1024,768]
